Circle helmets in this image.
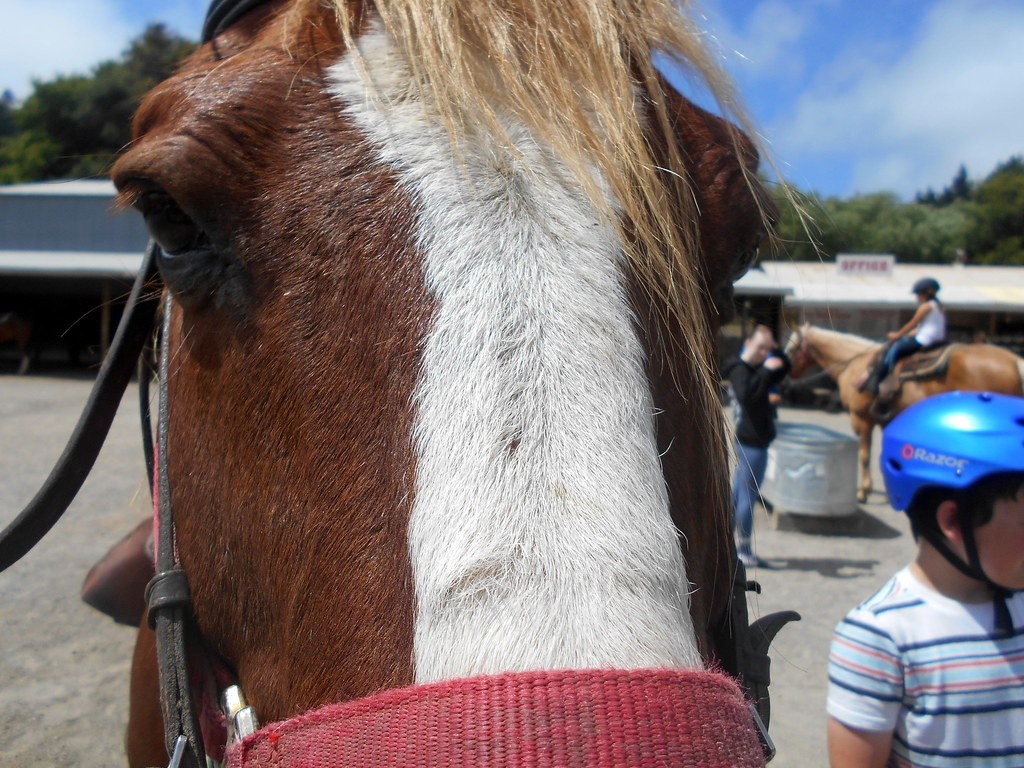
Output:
[880,390,1024,512]
[912,277,941,293]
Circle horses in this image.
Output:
[785,321,1024,503]
[78,2,822,767]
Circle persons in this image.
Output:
[726,323,793,566]
[858,278,943,393]
[824,392,1022,768]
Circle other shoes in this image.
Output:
[737,553,759,566]
